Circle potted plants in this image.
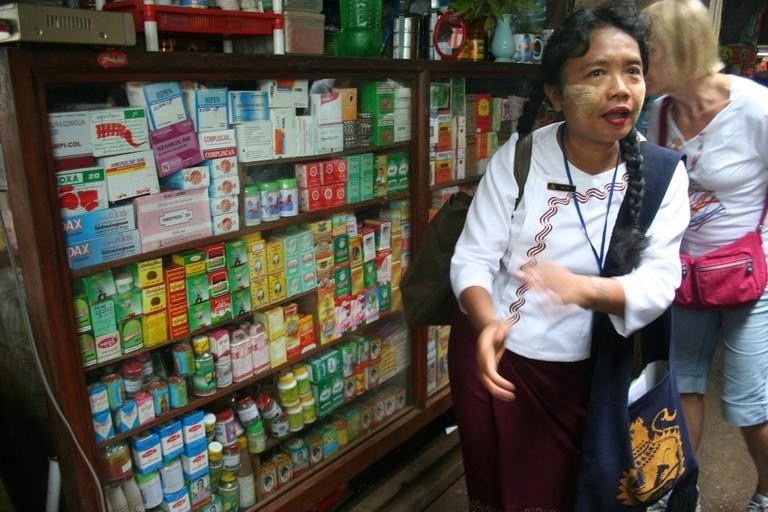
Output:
[447,0,538,63]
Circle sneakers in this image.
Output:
[645,483,702,512]
[743,492,768,512]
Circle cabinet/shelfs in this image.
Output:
[0,40,423,512]
[425,3,569,512]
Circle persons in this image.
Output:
[637,0,768,512]
[448,0,691,512]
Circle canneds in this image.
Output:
[120,359,143,398]
[132,350,155,383]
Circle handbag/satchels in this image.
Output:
[396,189,475,330]
[567,308,700,512]
[672,228,768,313]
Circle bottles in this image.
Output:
[393,13,427,60]
[123,361,143,398]
[285,403,303,432]
[215,410,237,446]
[208,441,223,492]
[256,394,280,421]
[245,185,261,226]
[223,441,242,472]
[276,371,300,407]
[258,180,281,222]
[275,177,298,217]
[299,392,318,425]
[338,0,383,58]
[236,395,261,427]
[247,421,266,454]
[291,362,310,396]
[218,473,239,512]
[239,436,258,509]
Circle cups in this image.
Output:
[514,33,542,64]
